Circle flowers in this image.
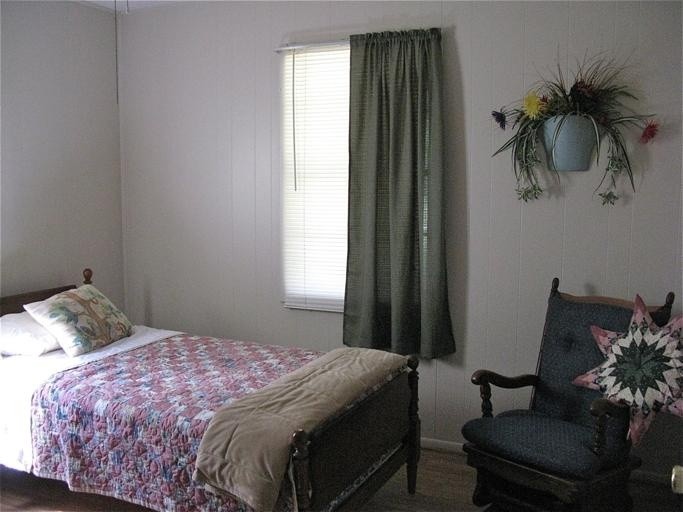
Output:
[490,44,658,206]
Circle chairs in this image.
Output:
[461,278,674,512]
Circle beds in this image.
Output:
[0,267,422,512]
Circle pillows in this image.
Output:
[0,311,60,357]
[22,284,135,358]
[572,293,683,446]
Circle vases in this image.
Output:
[539,116,606,172]
